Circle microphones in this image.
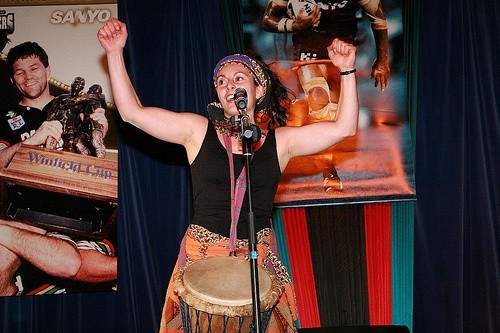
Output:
[234,87,248,109]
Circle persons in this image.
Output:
[262,0,390,193]
[96,17,358,333]
[0,40,109,295]
[0,218,117,295]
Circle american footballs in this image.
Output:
[287,0,317,20]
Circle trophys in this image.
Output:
[0,77,117,240]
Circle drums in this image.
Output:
[171,255,285,333]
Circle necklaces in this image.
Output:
[212,117,266,141]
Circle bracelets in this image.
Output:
[278,17,287,32]
[286,19,295,31]
[338,68,356,75]
